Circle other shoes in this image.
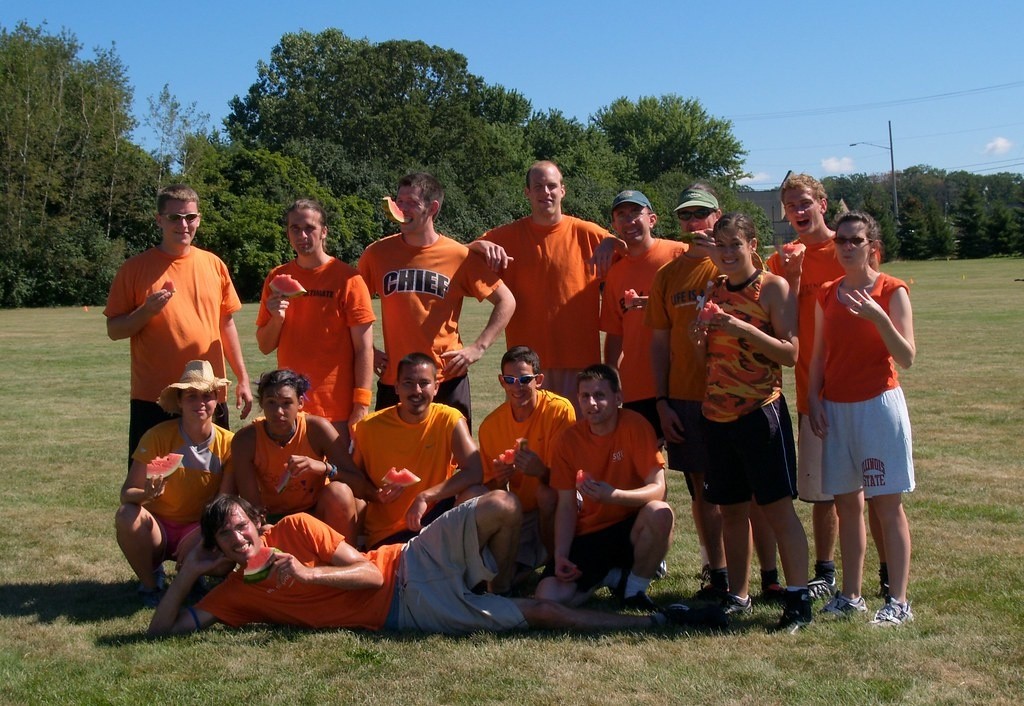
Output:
[184,571,208,602]
[138,566,163,604]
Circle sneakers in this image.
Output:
[815,590,867,621]
[767,588,813,639]
[609,570,629,597]
[662,602,728,634]
[688,580,727,603]
[623,591,655,613]
[807,576,839,602]
[760,579,785,599]
[876,577,892,601]
[867,599,914,629]
[716,592,753,618]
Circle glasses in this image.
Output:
[677,208,715,221]
[833,237,867,247]
[501,374,540,386]
[162,211,202,223]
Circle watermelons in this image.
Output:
[269,274,307,298]
[499,438,528,465]
[575,469,597,488]
[146,453,184,480]
[624,289,641,309]
[161,281,176,293]
[380,467,421,488]
[243,546,277,583]
[698,300,726,327]
[383,196,411,224]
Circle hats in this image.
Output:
[156,360,232,413]
[610,190,653,212]
[673,189,720,211]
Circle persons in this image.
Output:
[806,212,916,625]
[537,364,675,608]
[478,346,576,585]
[687,213,813,634]
[149,488,730,636]
[114,361,236,598]
[258,200,377,450]
[358,172,516,433]
[643,183,785,599]
[600,190,707,581]
[231,370,367,548]
[766,175,893,602]
[352,353,489,550]
[466,161,626,417]
[102,185,253,471]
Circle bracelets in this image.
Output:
[324,462,337,478]
[353,388,373,406]
[656,396,668,403]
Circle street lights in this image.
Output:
[850,142,898,218]
